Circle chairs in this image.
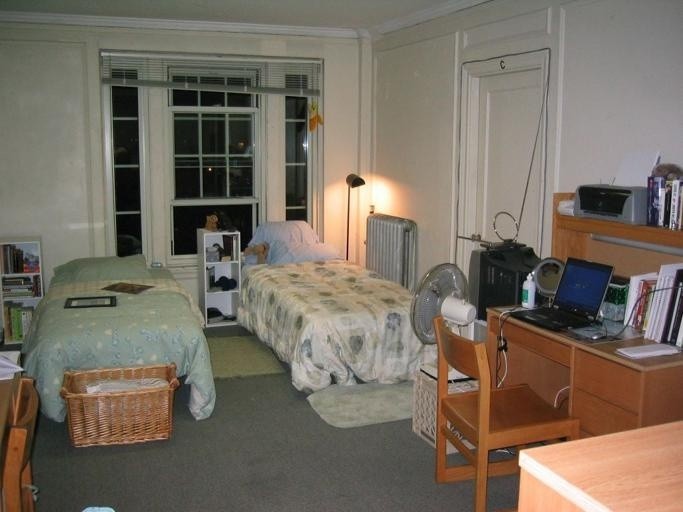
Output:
[432,316,580,512]
[0,378,39,512]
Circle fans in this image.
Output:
[409,263,477,384]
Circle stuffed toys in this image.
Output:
[215,276,236,291]
[206,243,224,261]
[206,214,219,232]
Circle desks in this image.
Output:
[0,350,22,455]
[515,421,683,512]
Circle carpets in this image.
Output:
[306,380,415,429]
[207,335,286,379]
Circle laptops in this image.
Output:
[509,256,615,333]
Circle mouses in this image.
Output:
[590,332,603,340]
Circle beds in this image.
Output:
[235,220,423,394]
[18,254,217,423]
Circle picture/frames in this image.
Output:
[65,295,117,308]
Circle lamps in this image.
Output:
[346,174,365,262]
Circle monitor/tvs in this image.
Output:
[466,246,542,322]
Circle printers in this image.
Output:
[573,183,647,226]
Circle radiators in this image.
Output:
[364,213,418,295]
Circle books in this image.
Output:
[624,263,683,347]
[647,176,683,230]
[222,234,237,261]
[0,244,41,343]
[207,267,215,292]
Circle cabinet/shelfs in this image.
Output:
[196,228,242,328]
[0,235,45,352]
[486,193,683,441]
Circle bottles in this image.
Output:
[520,273,536,308]
[205,266,210,291]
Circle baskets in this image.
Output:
[59,363,180,449]
[413,373,478,455]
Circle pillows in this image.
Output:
[267,239,341,264]
[49,254,151,286]
